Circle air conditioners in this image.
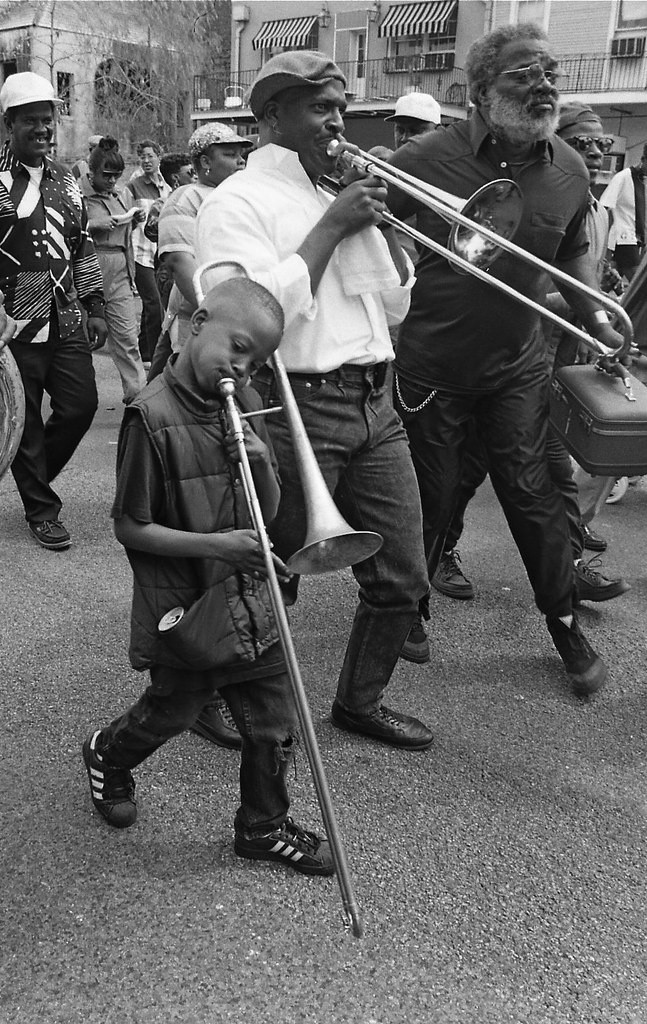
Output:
[609,35,646,58]
[601,155,622,171]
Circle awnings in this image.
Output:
[252,16,318,50]
[378,0,458,37]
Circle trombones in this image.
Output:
[324,138,633,360]
[188,258,385,939]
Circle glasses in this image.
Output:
[570,137,612,154]
[101,170,122,179]
[495,63,572,90]
[173,168,196,178]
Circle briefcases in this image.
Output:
[548,358,647,475]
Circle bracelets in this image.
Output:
[376,213,395,231]
[584,310,610,331]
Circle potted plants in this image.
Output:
[405,76,420,94]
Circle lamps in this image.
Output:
[366,2,381,22]
[318,8,331,28]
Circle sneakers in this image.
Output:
[400,616,431,662]
[82,726,138,828]
[573,559,633,601]
[30,520,73,549]
[235,816,335,876]
[431,549,477,597]
[546,610,607,695]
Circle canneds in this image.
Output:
[158,606,184,631]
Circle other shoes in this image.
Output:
[580,522,607,550]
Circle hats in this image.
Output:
[0,71,64,115]
[558,101,603,129]
[188,122,254,159]
[384,92,442,125]
[250,50,347,122]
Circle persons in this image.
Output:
[70,122,253,406]
[0,72,109,551]
[429,101,647,607]
[83,277,337,876]
[189,22,632,752]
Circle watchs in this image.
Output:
[339,176,347,188]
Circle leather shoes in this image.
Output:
[331,697,435,750]
[191,700,242,749]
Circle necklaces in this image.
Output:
[509,139,537,165]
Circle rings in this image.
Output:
[111,225,114,228]
